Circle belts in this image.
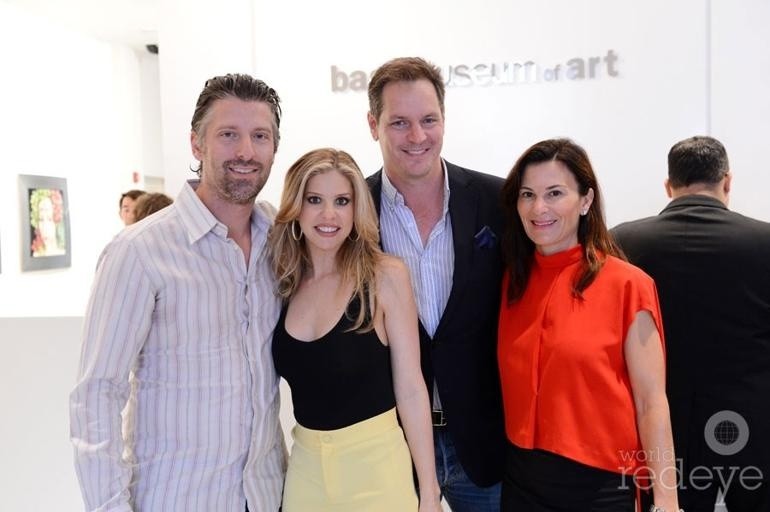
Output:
[430,410,446,428]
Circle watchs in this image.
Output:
[649,504,685,512]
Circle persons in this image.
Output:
[68,74,290,512]
[265,147,443,512]
[364,56,507,512]
[606,135,770,512]
[492,138,680,512]
[118,189,147,225]
[30,189,65,257]
